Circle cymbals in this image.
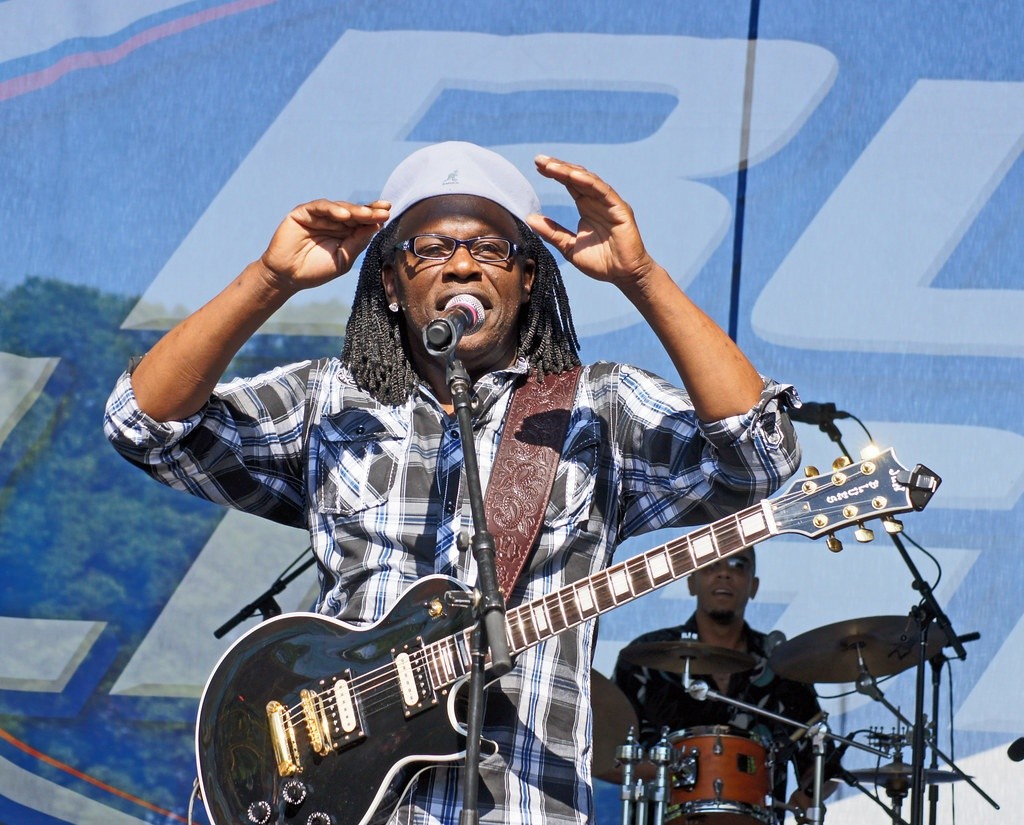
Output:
[829,763,975,783]
[590,667,638,776]
[768,616,946,684]
[620,640,758,675]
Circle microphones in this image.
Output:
[1007,737,1024,762]
[428,294,486,345]
[806,733,855,798]
[787,404,851,425]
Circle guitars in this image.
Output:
[195,448,942,825]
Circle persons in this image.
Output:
[608,544,839,825]
[102,140,803,825]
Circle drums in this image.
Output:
[654,723,777,825]
[596,720,658,785]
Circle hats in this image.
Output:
[379,141,540,238]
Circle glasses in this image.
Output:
[706,558,756,573]
[394,233,526,262]
